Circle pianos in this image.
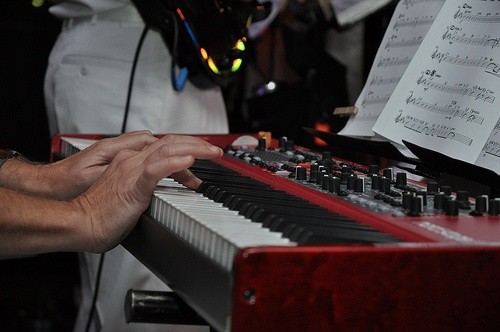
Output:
[48,132,500,332]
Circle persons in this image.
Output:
[1,131,223,262]
[44,0,229,332]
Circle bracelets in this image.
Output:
[0,149,24,168]
